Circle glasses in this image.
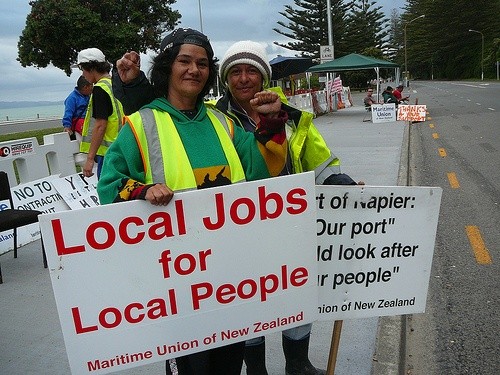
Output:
[369,91,373,92]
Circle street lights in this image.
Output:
[469,30,484,82]
[405,15,424,86]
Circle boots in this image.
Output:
[243,336,268,375]
[282,332,327,375]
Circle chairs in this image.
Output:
[363,97,374,122]
[0,171,47,284]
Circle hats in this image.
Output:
[396,85,406,88]
[71,48,106,68]
[160,28,214,59]
[219,41,272,89]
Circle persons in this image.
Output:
[364,87,381,111]
[111,40,365,375]
[62,75,93,141]
[70,48,125,181]
[392,85,409,102]
[382,86,401,108]
[96,27,288,375]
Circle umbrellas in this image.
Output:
[269,55,312,96]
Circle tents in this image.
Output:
[305,52,400,117]
